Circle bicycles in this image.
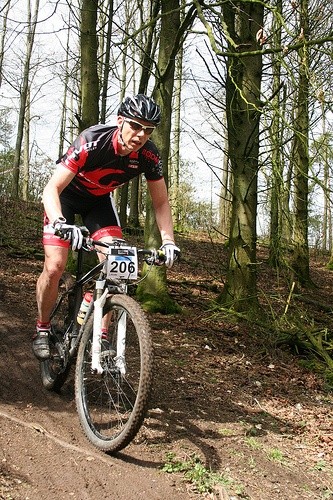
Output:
[40,227,166,454]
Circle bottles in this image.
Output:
[77,290,97,326]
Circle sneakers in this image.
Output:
[101,339,109,351]
[32,328,50,359]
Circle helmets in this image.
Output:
[118,93,161,128]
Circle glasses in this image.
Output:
[123,117,156,135]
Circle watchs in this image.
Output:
[52,217,67,229]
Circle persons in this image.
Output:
[32,94,181,362]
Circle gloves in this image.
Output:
[55,223,89,251]
[158,240,181,268]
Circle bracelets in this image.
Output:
[162,238,175,243]
